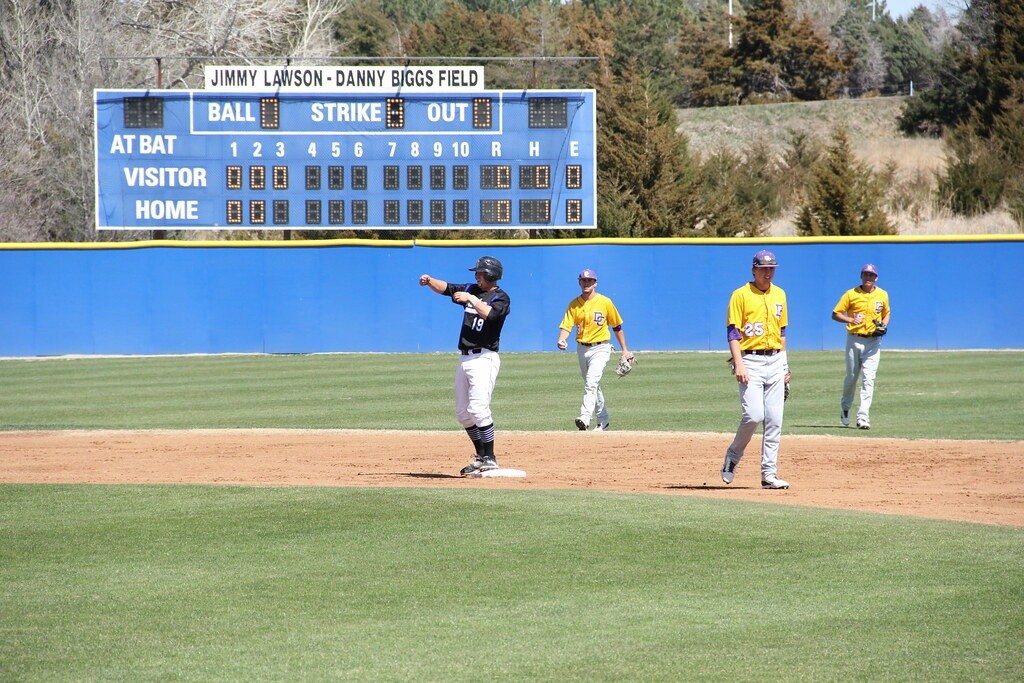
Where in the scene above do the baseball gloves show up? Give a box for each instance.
[613,351,636,378]
[784,381,791,403]
[871,318,887,337]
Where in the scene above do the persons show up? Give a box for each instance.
[419,256,510,478]
[557,268,635,431]
[831,264,891,430]
[720,249,792,490]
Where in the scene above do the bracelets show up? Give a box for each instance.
[474,300,480,306]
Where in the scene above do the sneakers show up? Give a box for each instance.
[841,409,850,425]
[721,453,736,484]
[761,476,789,489]
[857,418,870,429]
[460,455,484,475]
[480,456,499,472]
[593,422,609,431]
[575,416,589,431]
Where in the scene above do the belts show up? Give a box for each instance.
[744,349,780,356]
[578,342,601,346]
[462,349,481,355]
[851,333,872,338]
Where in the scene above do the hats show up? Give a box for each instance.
[861,264,878,277]
[577,269,597,285]
[752,250,779,268]
[469,256,502,282]
